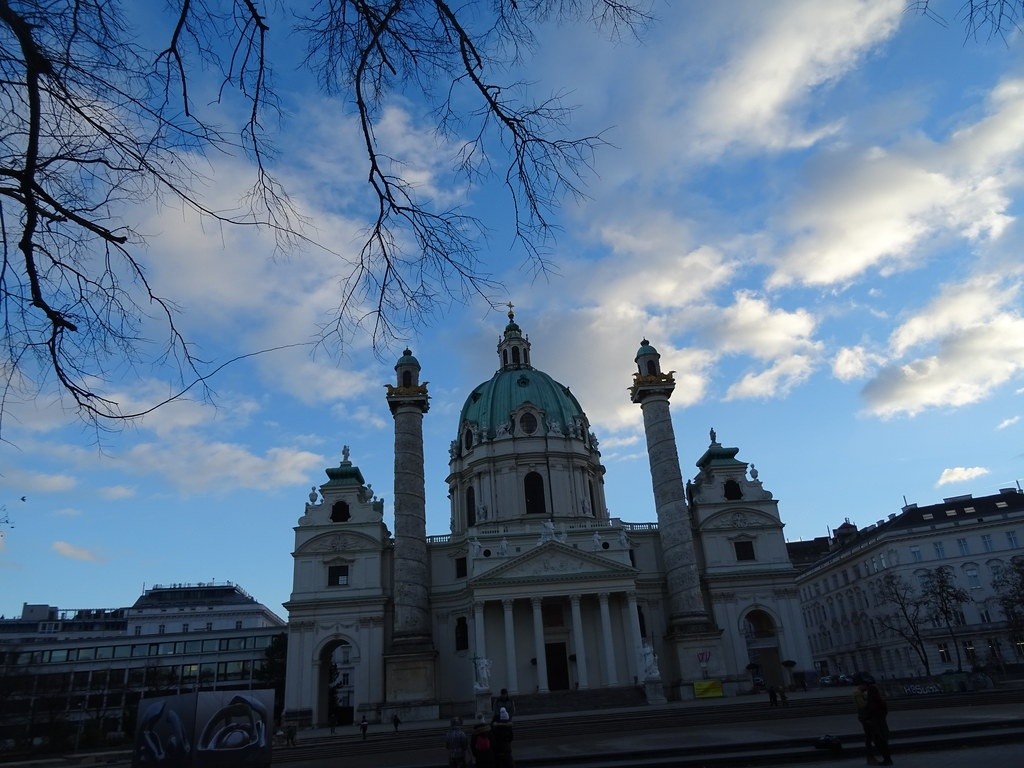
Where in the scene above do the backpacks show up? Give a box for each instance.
[475,735,489,750]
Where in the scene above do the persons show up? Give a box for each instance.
[768,686,777,707]
[393,715,401,732]
[492,688,514,722]
[329,714,336,735]
[286,721,296,746]
[470,713,497,768]
[360,716,368,740]
[801,679,809,692]
[445,716,467,768]
[852,671,893,766]
[491,706,514,768]
[779,683,791,707]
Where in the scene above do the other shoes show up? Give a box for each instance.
[867,756,893,767]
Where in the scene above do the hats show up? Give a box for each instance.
[451,717,461,726]
[472,713,485,725]
[499,707,509,720]
